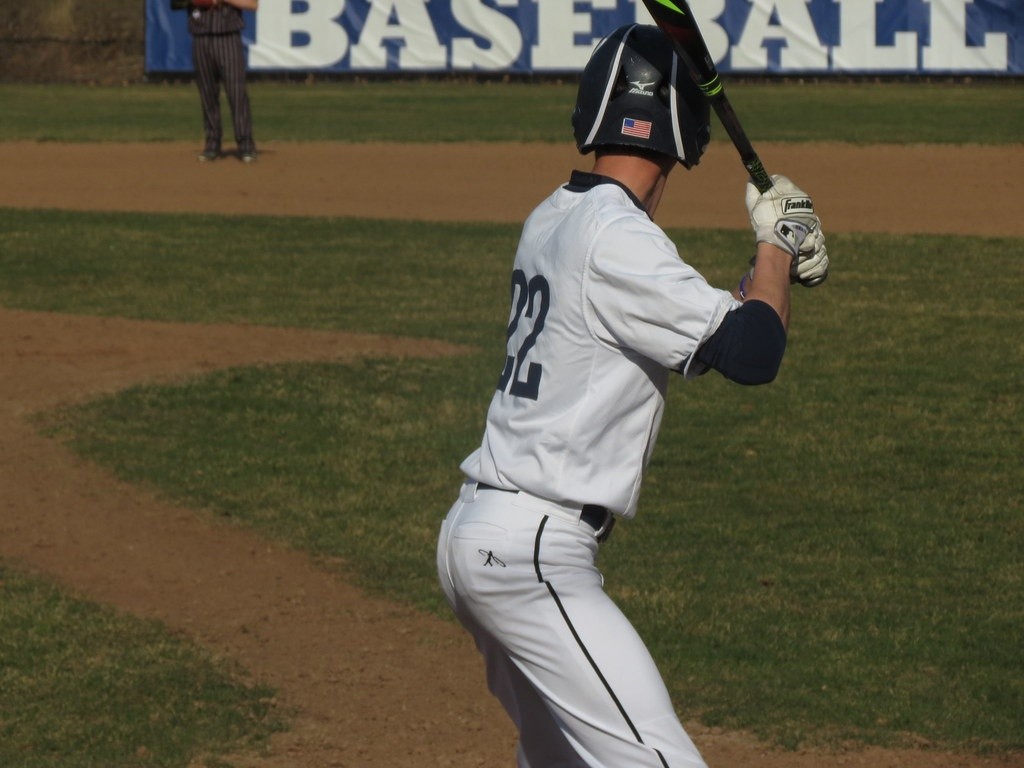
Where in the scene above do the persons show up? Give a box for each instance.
[426,14,835,767]
[173,1,269,164]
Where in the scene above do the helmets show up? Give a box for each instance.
[571,21,711,169]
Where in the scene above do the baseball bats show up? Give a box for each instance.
[643,0,775,196]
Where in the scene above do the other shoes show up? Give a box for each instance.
[238,149,257,162]
[199,150,221,162]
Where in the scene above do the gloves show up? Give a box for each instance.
[745,173,814,257]
[789,215,829,281]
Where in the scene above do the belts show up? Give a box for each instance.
[475,482,617,543]
